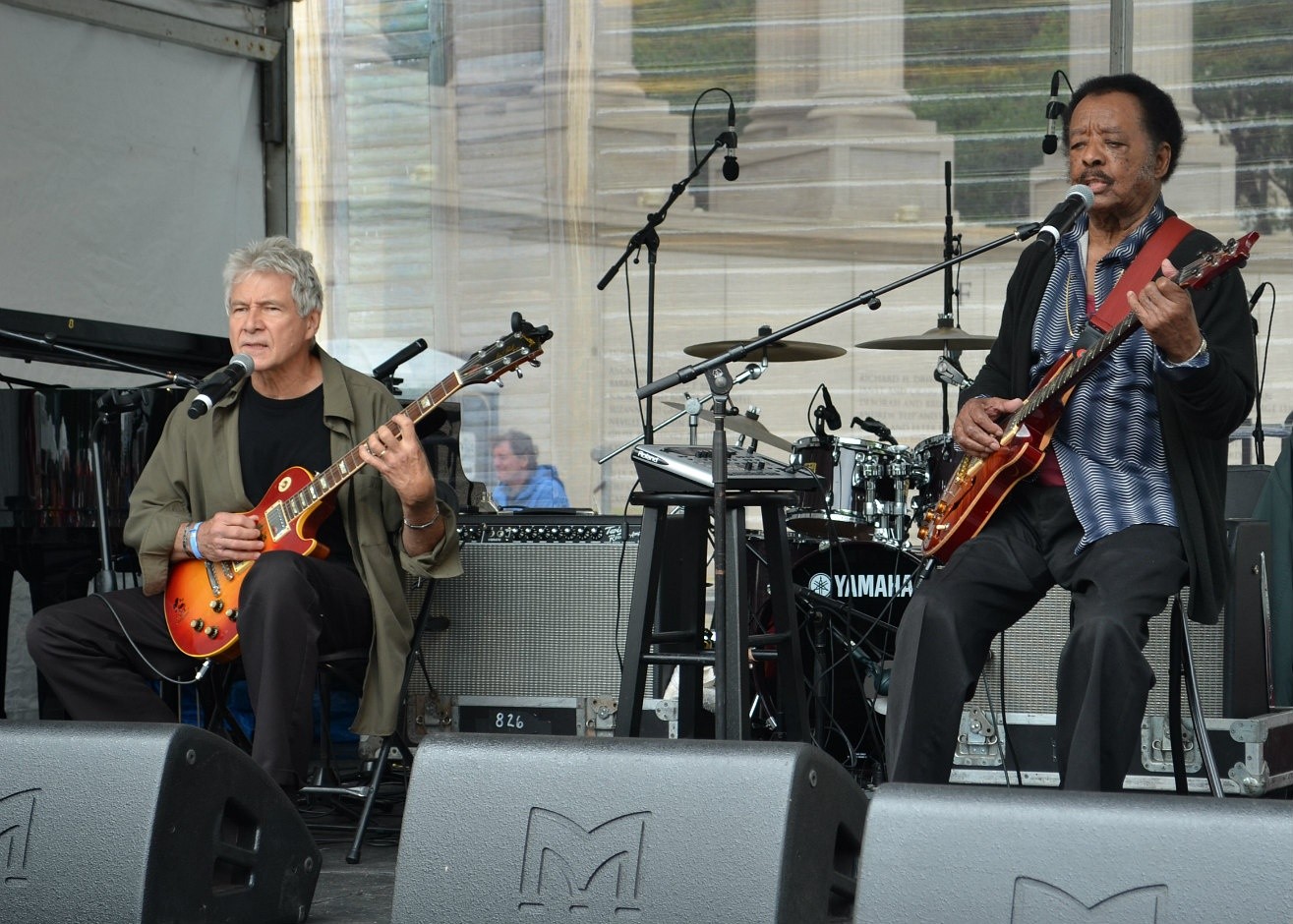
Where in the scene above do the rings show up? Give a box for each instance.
[378,449,387,457]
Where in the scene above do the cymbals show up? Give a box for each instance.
[855,327,999,350]
[683,336,847,362]
[661,401,794,453]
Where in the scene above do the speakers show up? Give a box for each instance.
[0,719,322,924]
[392,506,1293,924]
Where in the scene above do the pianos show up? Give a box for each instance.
[0,307,502,733]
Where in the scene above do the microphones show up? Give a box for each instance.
[822,386,842,430]
[1042,72,1060,155]
[1036,184,1095,254]
[1248,282,1266,312]
[872,668,892,695]
[723,102,741,181]
[185,352,255,418]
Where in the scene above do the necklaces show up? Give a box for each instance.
[1066,271,1075,337]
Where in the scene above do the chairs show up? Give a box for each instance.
[202,481,461,889]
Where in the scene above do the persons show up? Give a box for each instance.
[490,432,568,511]
[25,228,464,795]
[886,74,1257,790]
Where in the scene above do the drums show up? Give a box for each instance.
[912,433,966,529]
[710,529,823,634]
[785,436,912,541]
[750,541,926,759]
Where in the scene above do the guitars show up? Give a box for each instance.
[163,311,554,666]
[918,230,1261,566]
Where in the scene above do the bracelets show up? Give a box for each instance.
[1168,337,1206,365]
[404,504,439,529]
[183,523,192,557]
[189,522,205,559]
